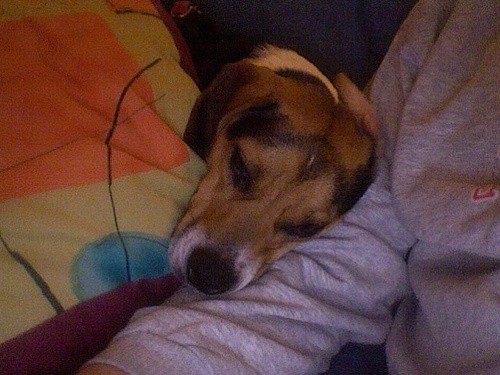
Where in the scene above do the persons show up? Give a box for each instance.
[75,0,500,373]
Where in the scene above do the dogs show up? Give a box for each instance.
[167,41,385,299]
